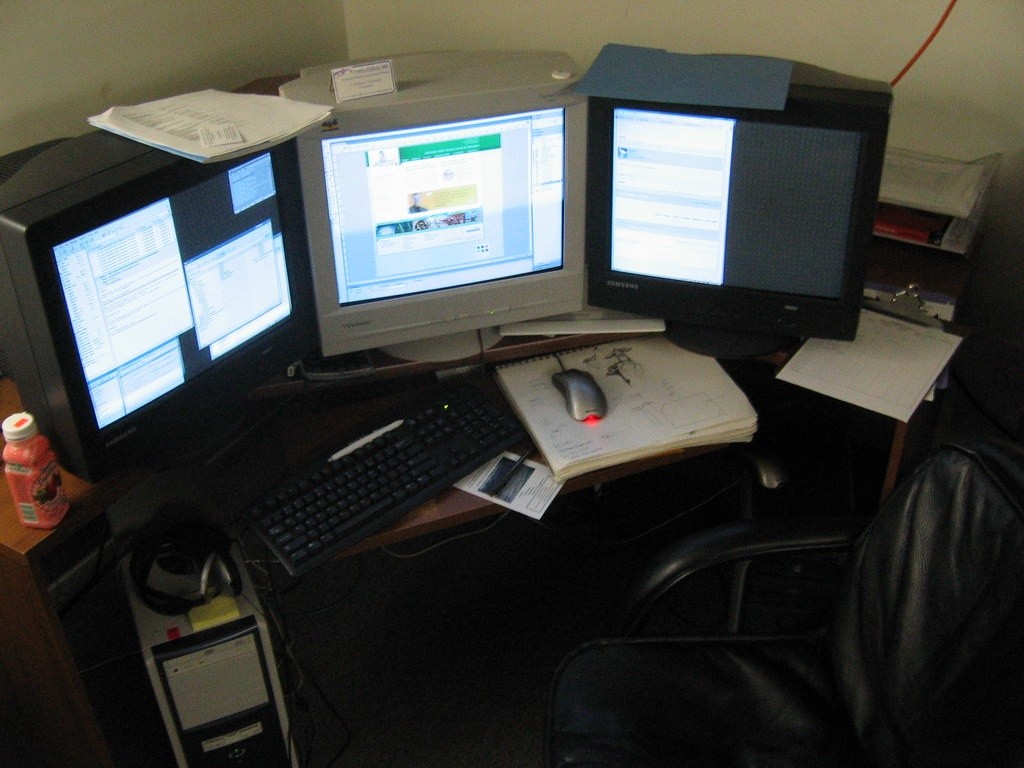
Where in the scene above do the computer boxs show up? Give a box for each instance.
[117,525,300,768]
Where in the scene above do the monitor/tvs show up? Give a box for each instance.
[276,48,588,362]
[0,129,318,486]
[584,55,894,361]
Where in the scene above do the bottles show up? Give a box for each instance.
[2,414,69,529]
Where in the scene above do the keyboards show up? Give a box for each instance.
[243,384,528,578]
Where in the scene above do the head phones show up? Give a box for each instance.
[128,525,244,617]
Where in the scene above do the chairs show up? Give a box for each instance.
[541,443,1024,768]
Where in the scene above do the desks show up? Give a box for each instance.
[0,306,919,768]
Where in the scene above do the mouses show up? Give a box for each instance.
[551,369,608,422]
